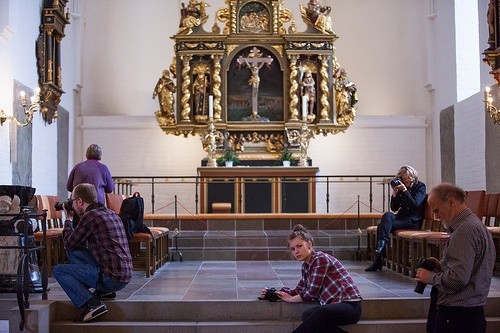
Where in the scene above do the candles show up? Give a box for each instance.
[302,96,307,117]
[209,95,213,118]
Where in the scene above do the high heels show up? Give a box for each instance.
[365,255,382,272]
[374,240,387,255]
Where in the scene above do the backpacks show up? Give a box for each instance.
[119,192,145,233]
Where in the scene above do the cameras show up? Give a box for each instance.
[55,198,73,211]
[390,177,404,188]
[414,258,436,295]
[260,288,282,302]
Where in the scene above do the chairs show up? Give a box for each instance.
[33,193,169,278]
[366,190,500,278]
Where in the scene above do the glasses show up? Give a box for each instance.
[69,197,81,206]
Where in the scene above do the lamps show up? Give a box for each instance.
[0,88,40,127]
[485,87,500,125]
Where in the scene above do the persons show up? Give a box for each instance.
[303,70,316,114]
[365,165,426,272]
[242,58,268,98]
[194,74,210,115]
[336,69,359,124]
[152,70,176,118]
[67,144,115,228]
[259,224,362,333]
[52,183,133,323]
[414,183,496,333]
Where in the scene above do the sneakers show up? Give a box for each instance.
[91,289,116,300]
[73,302,108,323]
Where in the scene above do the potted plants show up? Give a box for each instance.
[218,146,240,167]
[278,147,292,167]
